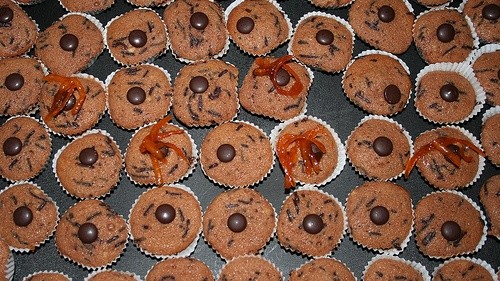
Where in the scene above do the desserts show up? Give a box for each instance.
[0,0,500,281]
